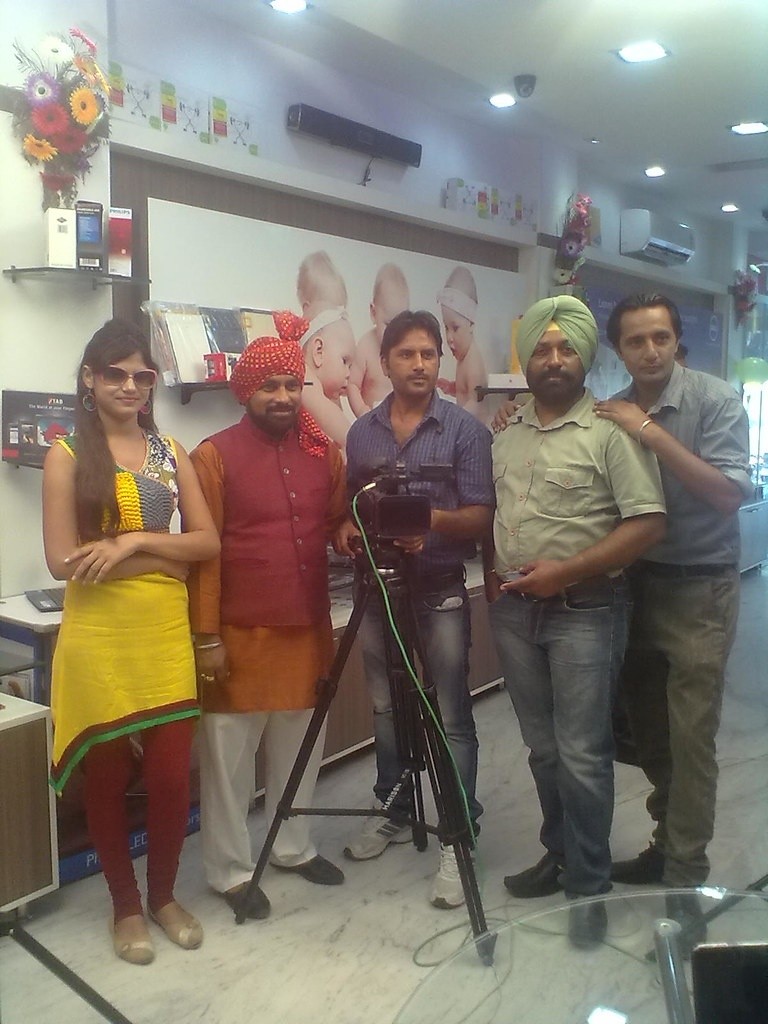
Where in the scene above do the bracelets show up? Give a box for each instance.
[196,642,223,649]
[638,419,653,448]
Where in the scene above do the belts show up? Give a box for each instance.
[497,577,564,602]
[634,560,733,577]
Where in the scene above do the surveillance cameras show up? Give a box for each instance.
[515,74,537,98]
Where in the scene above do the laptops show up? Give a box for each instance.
[25,588,67,612]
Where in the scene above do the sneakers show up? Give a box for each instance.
[430,836,480,909]
[345,798,414,860]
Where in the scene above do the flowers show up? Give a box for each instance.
[10,26,112,212]
[553,192,593,284]
[728,268,763,327]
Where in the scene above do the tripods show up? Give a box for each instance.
[234,533,499,966]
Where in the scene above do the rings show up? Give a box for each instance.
[201,673,214,681]
[227,672,231,679]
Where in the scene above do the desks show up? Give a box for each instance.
[0,588,65,705]
[393,887,768,1024]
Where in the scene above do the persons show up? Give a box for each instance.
[481,295,668,949]
[436,266,489,424]
[297,251,410,449]
[185,337,348,920]
[490,294,755,959]
[331,309,497,910]
[41,317,222,965]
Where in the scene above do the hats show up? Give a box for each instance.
[516,296,599,380]
[229,312,331,457]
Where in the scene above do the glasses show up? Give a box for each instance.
[100,363,158,390]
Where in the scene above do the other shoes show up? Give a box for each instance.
[503,865,565,898]
[268,856,345,884]
[570,894,607,947]
[222,880,269,918]
[147,897,202,949]
[609,851,664,885]
[114,914,155,964]
[666,887,707,959]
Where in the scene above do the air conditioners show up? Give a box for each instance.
[620,208,696,266]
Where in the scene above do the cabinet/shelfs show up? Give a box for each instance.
[0,692,61,919]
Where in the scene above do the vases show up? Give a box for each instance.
[548,285,576,297]
